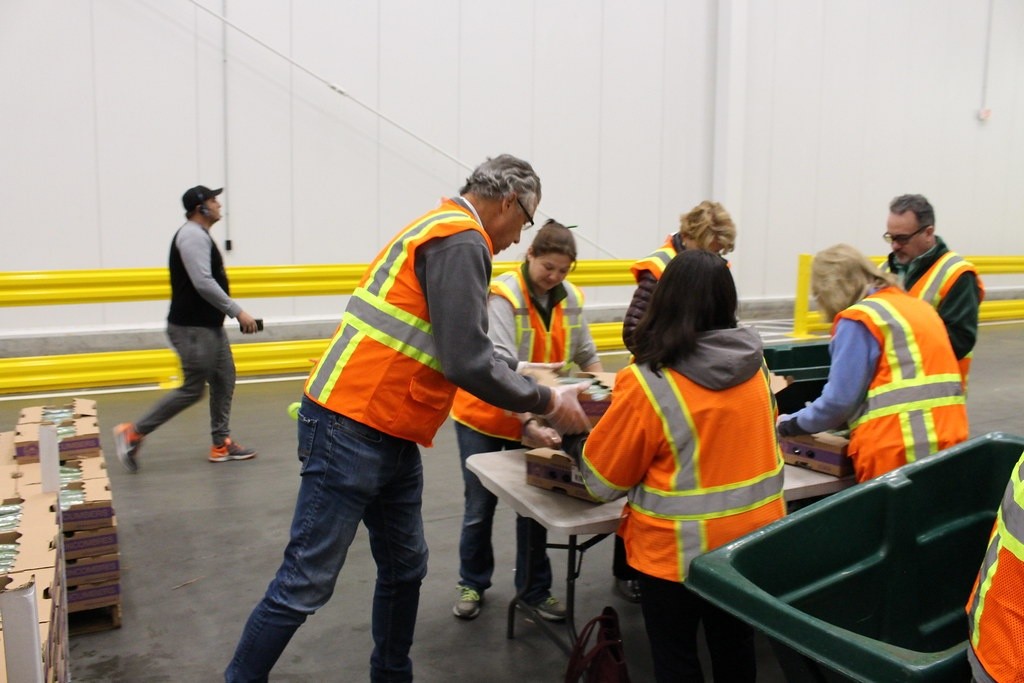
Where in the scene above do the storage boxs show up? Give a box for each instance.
[764,340,831,415]
[524,447,601,504]
[0,398,123,683]
[778,431,855,478]
[539,372,617,428]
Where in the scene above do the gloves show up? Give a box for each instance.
[561,431,589,466]
[778,416,809,438]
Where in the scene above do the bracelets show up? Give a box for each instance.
[536,386,563,419]
[522,416,537,431]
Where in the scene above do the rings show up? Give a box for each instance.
[551,437,558,441]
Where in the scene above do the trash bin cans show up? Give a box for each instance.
[687,427,1024,683]
[758,336,834,414]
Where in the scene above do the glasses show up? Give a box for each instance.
[883,224,932,244]
[517,198,534,230]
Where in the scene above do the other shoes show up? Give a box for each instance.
[615,577,642,603]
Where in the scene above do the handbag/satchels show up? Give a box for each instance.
[567,605,628,683]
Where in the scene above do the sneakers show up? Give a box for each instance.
[515,596,566,621]
[208,435,257,462]
[453,585,482,618]
[111,423,147,475]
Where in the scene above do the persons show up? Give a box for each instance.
[776,243,970,487]
[613,202,738,601]
[450,220,602,621]
[113,185,264,471]
[580,249,790,683]
[966,443,1023,681]
[221,154,594,683]
[873,194,987,410]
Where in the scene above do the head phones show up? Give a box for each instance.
[192,187,210,216]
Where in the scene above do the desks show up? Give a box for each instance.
[464,448,859,681]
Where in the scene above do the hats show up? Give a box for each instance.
[182,185,222,212]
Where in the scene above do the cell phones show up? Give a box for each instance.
[240,319,263,331]
